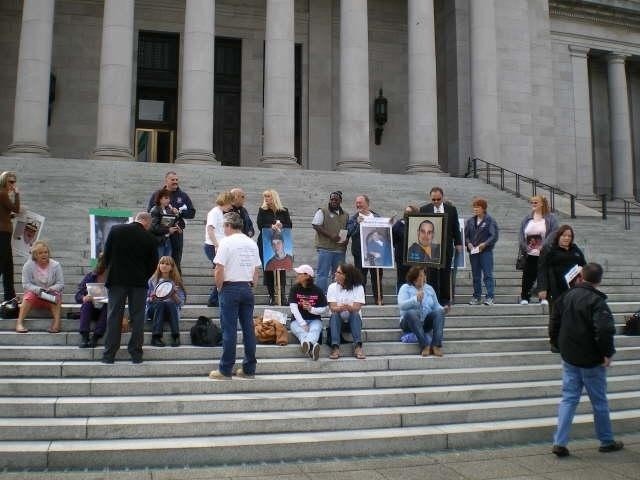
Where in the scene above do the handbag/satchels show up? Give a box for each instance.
[516,255,528,269]
[0,298,19,319]
[191,316,222,347]
[625,309,640,336]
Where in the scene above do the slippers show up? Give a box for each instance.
[48,327,60,333]
[16,328,28,332]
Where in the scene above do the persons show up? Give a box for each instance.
[548,263,624,456]
[348,195,396,305]
[367,229,392,266]
[203,189,293,378]
[0,171,20,301]
[408,220,440,262]
[397,264,448,356]
[465,196,498,305]
[75,170,196,365]
[311,190,349,294]
[16,241,65,332]
[392,205,418,293]
[419,186,462,308]
[288,265,330,361]
[520,195,560,305]
[327,264,366,359]
[537,225,588,353]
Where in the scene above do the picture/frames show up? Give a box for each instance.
[154,279,176,301]
[402,213,448,269]
[160,213,177,238]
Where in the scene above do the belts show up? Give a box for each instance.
[223,281,252,286]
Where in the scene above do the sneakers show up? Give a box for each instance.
[309,342,320,361]
[482,297,496,305]
[267,295,274,305]
[353,348,365,359]
[422,345,432,356]
[329,350,341,359]
[79,335,89,348]
[551,344,559,353]
[88,336,99,347]
[282,294,290,306]
[101,359,114,362]
[236,368,256,379]
[433,346,443,355]
[551,445,568,455]
[207,302,218,307]
[301,340,313,357]
[133,359,145,363]
[468,297,482,305]
[209,370,232,380]
[540,298,549,304]
[599,441,624,452]
[520,299,528,304]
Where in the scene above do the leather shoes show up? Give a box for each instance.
[171,337,180,347]
[151,335,165,347]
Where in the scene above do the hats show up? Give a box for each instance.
[292,264,314,278]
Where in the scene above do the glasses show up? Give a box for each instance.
[330,197,339,201]
[8,181,16,183]
[431,197,441,201]
[336,270,345,275]
[241,196,245,198]
[530,202,539,204]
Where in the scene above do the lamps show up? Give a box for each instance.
[374,87,388,143]
